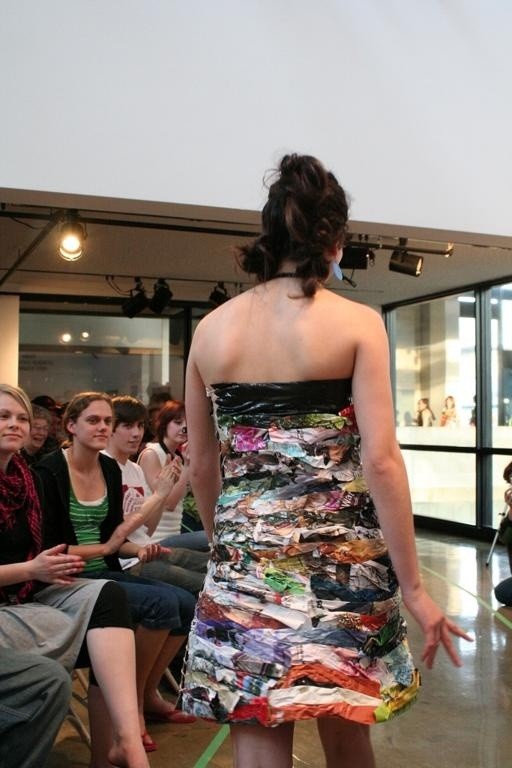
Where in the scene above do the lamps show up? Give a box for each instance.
[123,279,230,320]
[55,207,88,264]
[57,319,185,356]
[341,235,424,277]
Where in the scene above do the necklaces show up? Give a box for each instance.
[268,268,311,279]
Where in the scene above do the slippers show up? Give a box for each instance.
[143,709,197,724]
[141,732,157,752]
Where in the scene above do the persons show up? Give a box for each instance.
[438,395,458,429]
[173,149,477,767]
[492,459,512,608]
[416,397,435,427]
[1,381,211,767]
